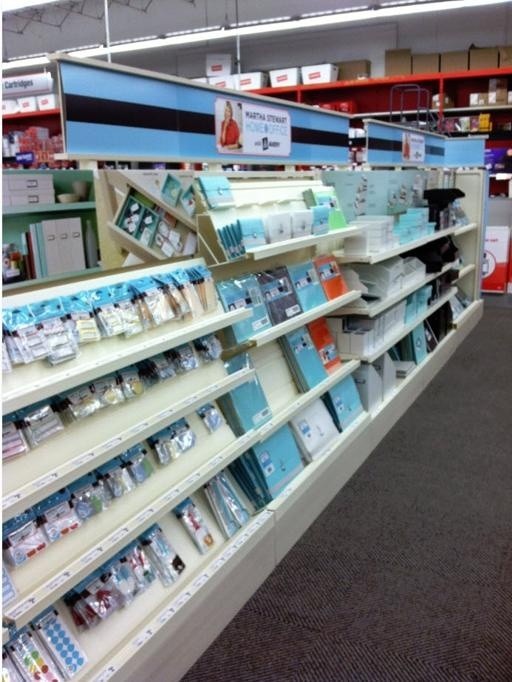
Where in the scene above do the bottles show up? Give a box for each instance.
[85,220,99,267]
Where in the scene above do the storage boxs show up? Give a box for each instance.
[301,44,512,86]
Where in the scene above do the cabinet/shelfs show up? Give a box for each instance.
[1,67,512,682]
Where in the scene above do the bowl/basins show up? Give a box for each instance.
[58,194,79,202]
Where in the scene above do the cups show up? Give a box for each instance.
[71,180,89,200]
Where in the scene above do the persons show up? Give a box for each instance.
[219,100,240,149]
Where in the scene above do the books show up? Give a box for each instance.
[215,254,367,512]
